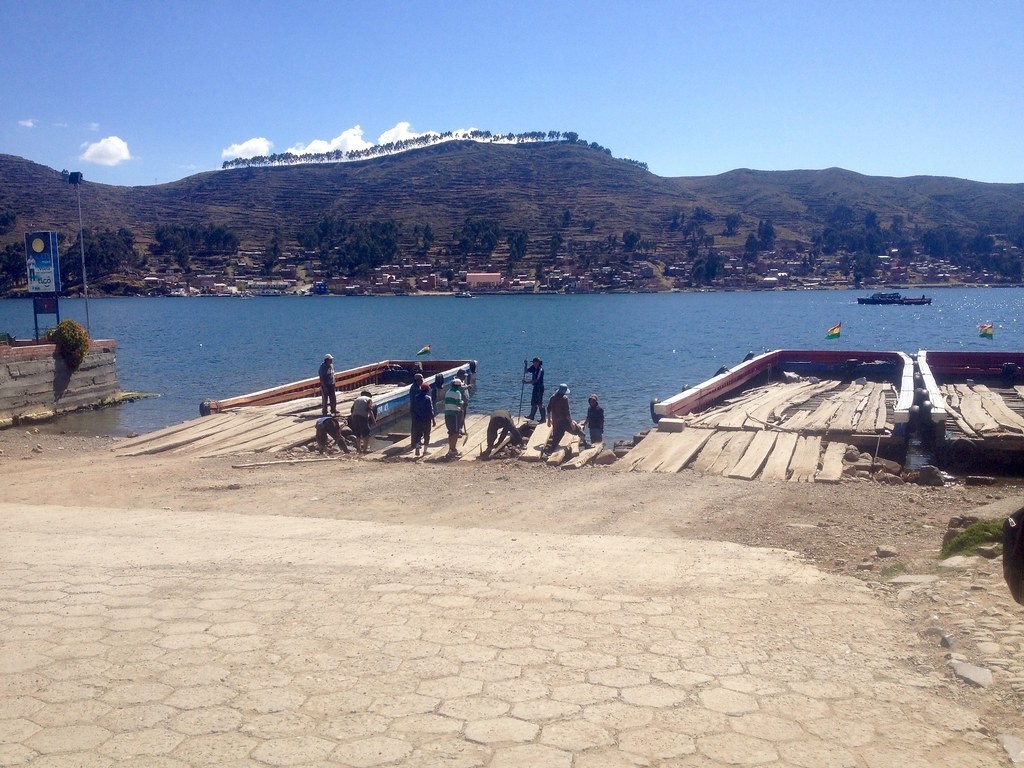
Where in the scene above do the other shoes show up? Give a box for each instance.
[520,444,527,449]
[481,450,491,457]
[448,449,461,456]
[459,430,467,438]
[342,447,351,453]
[546,447,553,455]
[584,442,594,448]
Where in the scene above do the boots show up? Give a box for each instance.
[539,407,546,422]
[524,405,536,420]
[416,442,420,455]
[424,445,431,455]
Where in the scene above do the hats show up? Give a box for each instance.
[420,383,432,391]
[556,383,571,394]
[324,354,334,360]
[530,358,542,363]
[457,369,469,377]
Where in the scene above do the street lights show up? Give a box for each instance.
[68,171,90,339]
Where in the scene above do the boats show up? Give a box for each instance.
[454,292,472,298]
[856,292,932,305]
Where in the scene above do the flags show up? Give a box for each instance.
[825,324,840,340]
[979,325,994,340]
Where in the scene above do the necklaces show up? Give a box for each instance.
[445,379,464,458]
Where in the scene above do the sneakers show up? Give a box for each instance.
[357,448,372,453]
[322,410,341,414]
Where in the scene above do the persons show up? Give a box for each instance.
[482,409,524,455]
[546,383,596,456]
[452,368,474,438]
[351,391,376,452]
[410,374,436,455]
[522,358,546,423]
[582,394,604,443]
[318,354,340,417]
[315,417,351,454]
